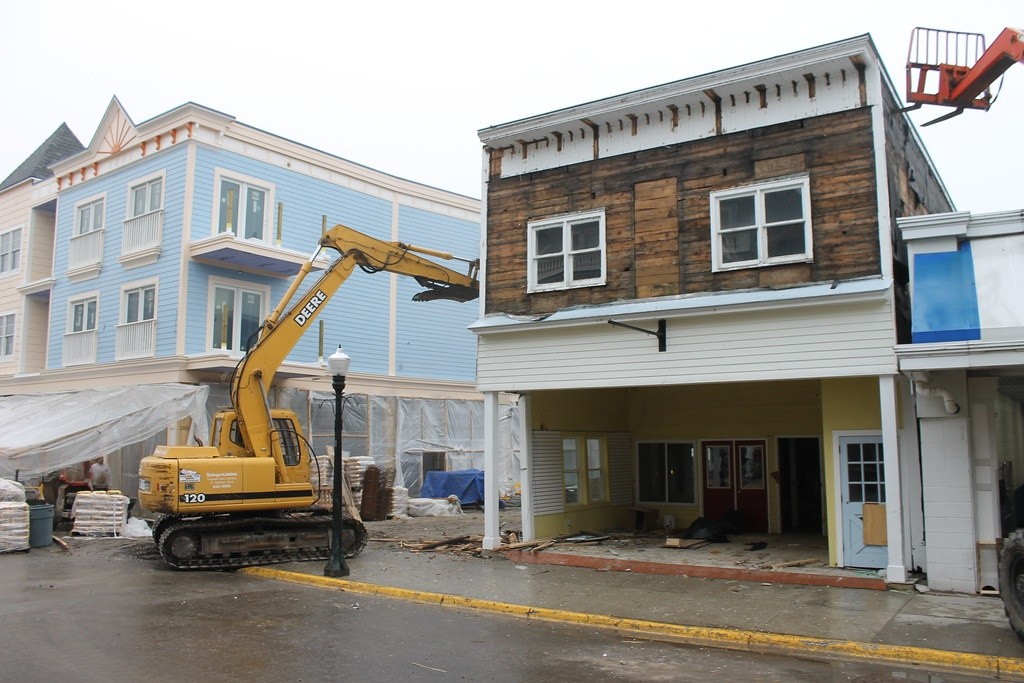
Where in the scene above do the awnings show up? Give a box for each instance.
[0,383,210,481]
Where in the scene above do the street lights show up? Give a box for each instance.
[323,341,349,581]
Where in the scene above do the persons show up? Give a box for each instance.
[88,457,112,491]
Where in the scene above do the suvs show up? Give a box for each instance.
[906,23,1024,638]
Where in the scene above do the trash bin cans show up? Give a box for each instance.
[28,504,55,547]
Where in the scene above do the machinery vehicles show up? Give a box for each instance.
[135,223,481,573]
